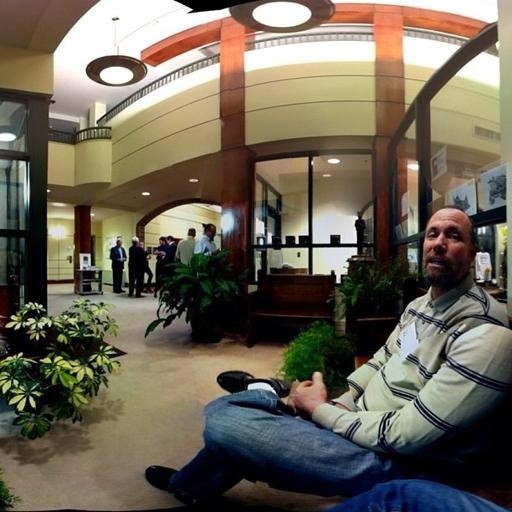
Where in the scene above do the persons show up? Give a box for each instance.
[110,240,128,293]
[144,206,512,507]
[323,478,512,512]
[128,224,216,298]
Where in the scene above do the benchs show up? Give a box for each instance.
[355,276,430,369]
[250,271,336,331]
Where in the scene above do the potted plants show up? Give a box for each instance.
[144,248,250,344]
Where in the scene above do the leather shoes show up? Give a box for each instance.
[217,371,282,395]
[145,465,192,506]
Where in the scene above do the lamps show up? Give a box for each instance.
[229,0,336,33]
[84,18,147,86]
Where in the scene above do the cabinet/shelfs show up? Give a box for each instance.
[74,269,103,295]
